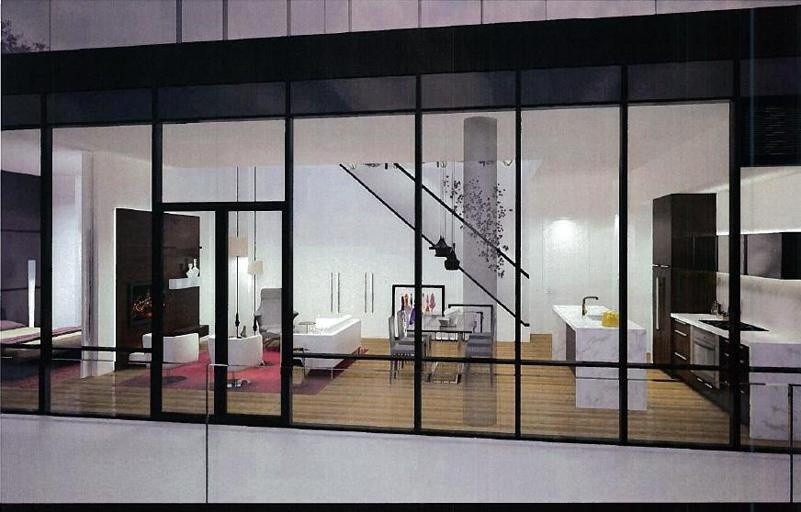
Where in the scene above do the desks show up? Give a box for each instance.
[297,319,317,332]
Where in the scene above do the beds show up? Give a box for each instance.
[0,317,82,365]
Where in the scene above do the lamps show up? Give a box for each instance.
[229,231,250,339]
[248,259,264,333]
[428,161,465,272]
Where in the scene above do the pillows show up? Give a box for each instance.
[0,318,25,330]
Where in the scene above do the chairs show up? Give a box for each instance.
[207,333,264,391]
[147,333,201,385]
[254,286,299,351]
[387,307,498,384]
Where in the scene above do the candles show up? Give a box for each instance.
[390,283,447,340]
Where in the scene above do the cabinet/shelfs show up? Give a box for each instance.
[650,192,720,379]
[718,336,752,428]
[672,319,689,384]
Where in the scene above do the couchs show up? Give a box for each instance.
[278,313,363,381]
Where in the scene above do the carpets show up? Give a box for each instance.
[113,343,367,398]
[0,355,87,391]
